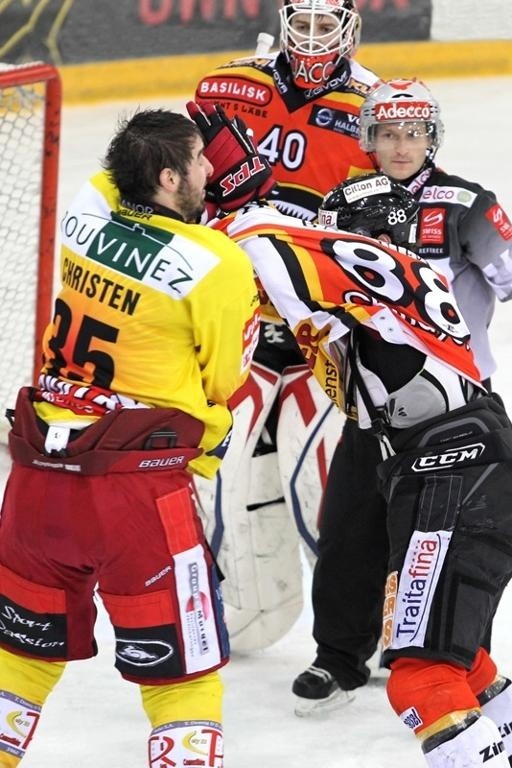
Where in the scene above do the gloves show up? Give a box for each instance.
[181,97,276,207]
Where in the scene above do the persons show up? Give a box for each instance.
[183,97,512,768]
[285,77,508,697]
[0,107,265,768]
[184,0,388,627]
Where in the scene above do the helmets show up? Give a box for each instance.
[357,75,446,153]
[279,0,363,88]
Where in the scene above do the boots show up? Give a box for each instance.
[294,656,352,702]
